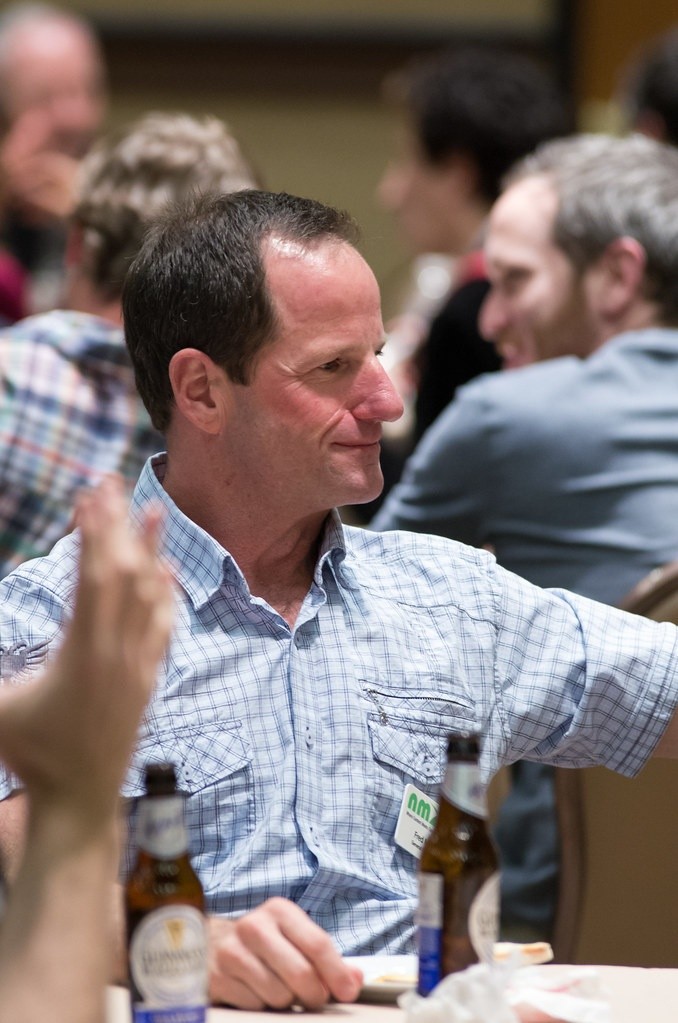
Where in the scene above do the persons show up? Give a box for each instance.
[0,1,678,1023]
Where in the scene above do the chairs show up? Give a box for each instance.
[550,560,678,968]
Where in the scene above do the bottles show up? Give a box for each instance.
[123,763,213,1023]
[417,729,499,998]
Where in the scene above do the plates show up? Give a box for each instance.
[343,955,417,1002]
